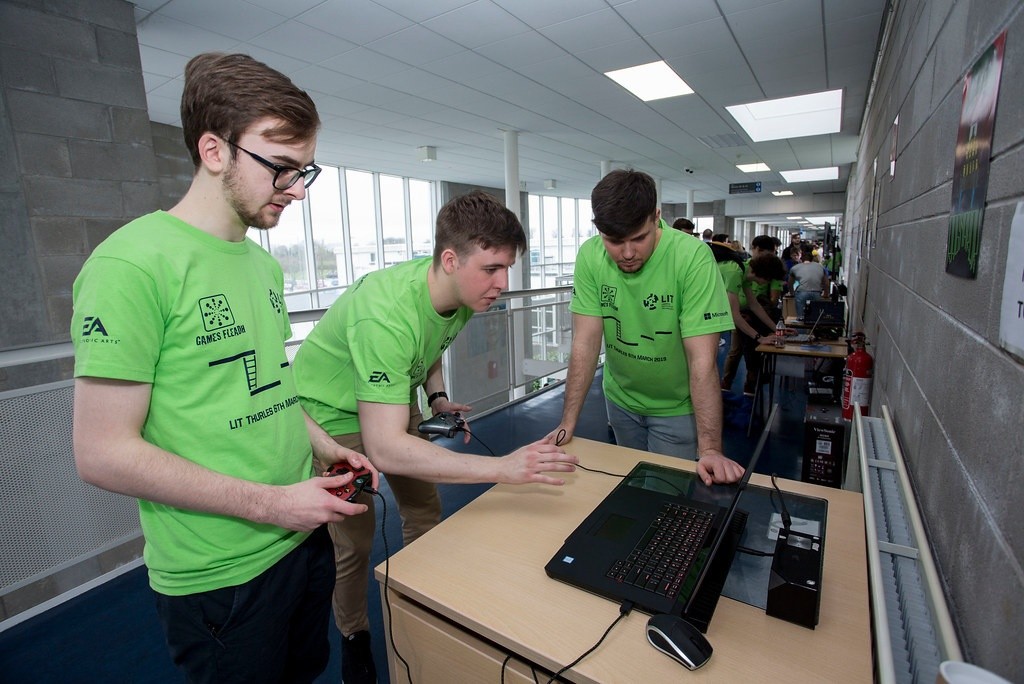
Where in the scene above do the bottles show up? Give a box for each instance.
[775,316,786,348]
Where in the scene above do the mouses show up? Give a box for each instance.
[647,614,714,671]
[773,341,784,348]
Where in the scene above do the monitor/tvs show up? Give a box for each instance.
[823,222,831,257]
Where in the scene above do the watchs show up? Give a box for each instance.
[754,334,762,341]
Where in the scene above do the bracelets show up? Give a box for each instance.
[428,392,448,407]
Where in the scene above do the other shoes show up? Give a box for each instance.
[720,380,732,393]
[744,384,754,397]
[718,338,726,347]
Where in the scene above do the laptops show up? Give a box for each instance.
[783,309,825,343]
[545,403,779,616]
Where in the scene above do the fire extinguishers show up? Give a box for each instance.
[842,331,874,420]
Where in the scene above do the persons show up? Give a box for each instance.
[672,218,842,397]
[542,169,746,486]
[290,189,579,684]
[71,52,379,684]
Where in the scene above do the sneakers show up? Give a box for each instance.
[341,630,381,684]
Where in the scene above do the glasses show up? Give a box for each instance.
[223,138,322,190]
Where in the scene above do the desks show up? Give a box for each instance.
[373,438,873,684]
[782,291,832,320]
[746,316,849,438]
